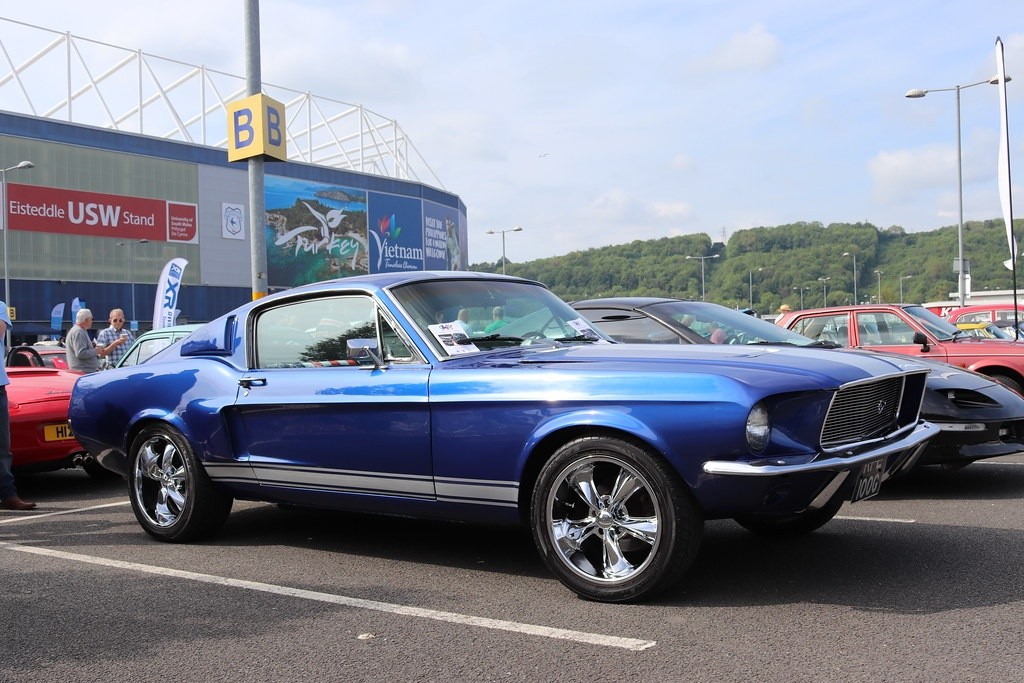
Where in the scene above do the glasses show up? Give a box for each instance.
[112,318,122,322]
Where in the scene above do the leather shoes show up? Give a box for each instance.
[0,497,37,509]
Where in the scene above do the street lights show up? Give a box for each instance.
[817,276,831,308]
[904,74,1014,309]
[899,275,912,304]
[874,269,883,304]
[686,254,721,302]
[485,225,523,276]
[0,159,35,355]
[794,287,810,311]
[842,252,857,306]
[748,268,764,310]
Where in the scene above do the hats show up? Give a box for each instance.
[777,305,792,312]
[60,336,65,340]
[743,310,756,314]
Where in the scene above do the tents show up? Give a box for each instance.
[11,323,62,336]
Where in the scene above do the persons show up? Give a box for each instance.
[0,301,38,510]
[485,306,510,333]
[65,309,105,373]
[455,308,473,338]
[444,214,460,271]
[57,336,65,348]
[774,304,800,332]
[96,309,134,366]
[707,321,726,344]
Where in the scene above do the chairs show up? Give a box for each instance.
[836,325,876,345]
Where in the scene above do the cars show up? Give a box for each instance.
[5,345,121,482]
[772,302,1024,399]
[483,296,1024,489]
[104,322,209,371]
[67,267,944,605]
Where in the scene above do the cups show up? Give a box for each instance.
[121,334,127,339]
[96,343,104,348]
